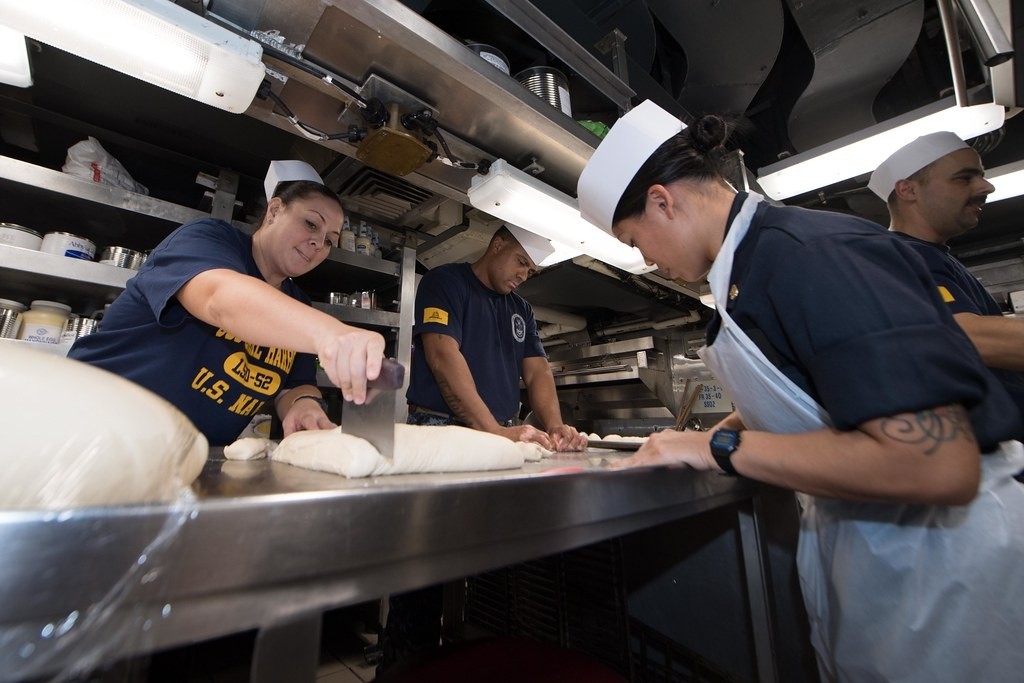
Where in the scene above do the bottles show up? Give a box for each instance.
[332,215,382,259]
[0,297,111,346]
[329,288,377,310]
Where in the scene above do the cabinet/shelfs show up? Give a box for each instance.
[1,153,417,424]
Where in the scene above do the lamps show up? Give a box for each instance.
[756,44,1024,204]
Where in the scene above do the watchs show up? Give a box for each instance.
[293,395,332,421]
[709,427,741,477]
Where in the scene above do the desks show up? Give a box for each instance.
[0,438,783,683]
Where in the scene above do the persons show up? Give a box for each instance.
[61,157,386,459]
[375,221,589,682]
[869,131,1024,484]
[577,99,1024,683]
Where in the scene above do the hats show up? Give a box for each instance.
[867,131,972,203]
[577,99,689,238]
[505,222,558,266]
[264,160,324,203]
